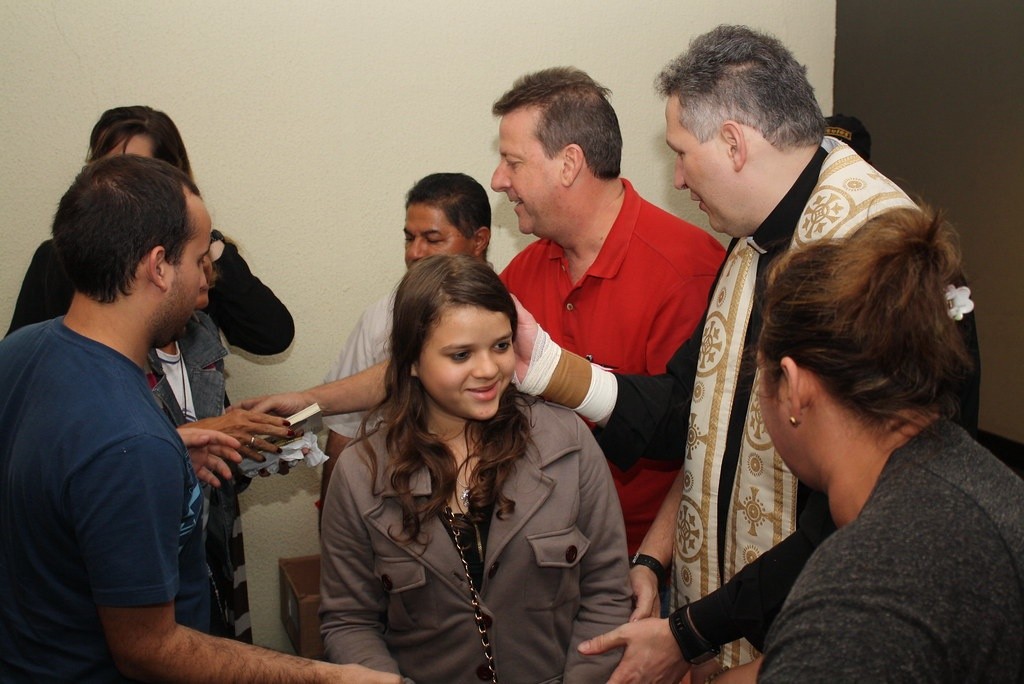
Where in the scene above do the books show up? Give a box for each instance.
[247,401,323,453]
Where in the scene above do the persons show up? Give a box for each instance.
[577,24,981,684]
[4,102,294,638]
[313,173,491,535]
[222,65,729,620]
[0,157,406,684]
[715,210,1024,684]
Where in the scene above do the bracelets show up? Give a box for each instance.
[629,552,667,591]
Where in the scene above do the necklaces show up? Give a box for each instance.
[458,478,470,507]
[179,355,187,416]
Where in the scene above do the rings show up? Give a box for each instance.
[250,437,254,444]
